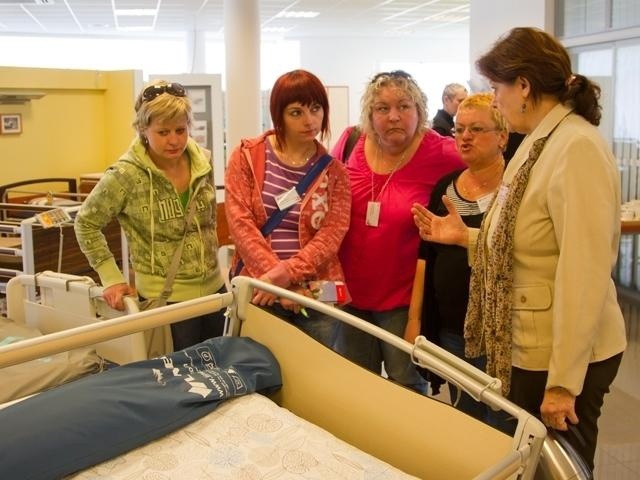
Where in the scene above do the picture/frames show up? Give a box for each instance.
[0,113,21,134]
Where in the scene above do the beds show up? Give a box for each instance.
[0,272,147,407]
[0,276,547,480]
[0,178,90,219]
[0,203,121,297]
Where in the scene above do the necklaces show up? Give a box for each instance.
[282,148,315,165]
[462,160,504,197]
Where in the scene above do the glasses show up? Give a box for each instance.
[142,83,187,101]
[371,70,411,83]
[451,126,500,135]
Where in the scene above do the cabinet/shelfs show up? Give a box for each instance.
[149,74,226,200]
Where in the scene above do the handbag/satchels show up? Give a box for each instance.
[229,154,333,284]
[133,149,211,358]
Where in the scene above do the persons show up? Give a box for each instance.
[224,70,352,350]
[412,27,624,476]
[403,94,513,436]
[329,71,469,396]
[424,83,468,151]
[73,79,227,354]
